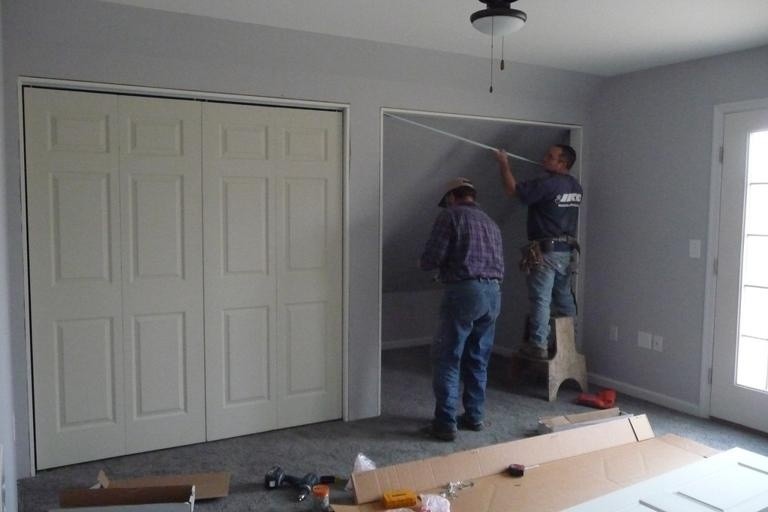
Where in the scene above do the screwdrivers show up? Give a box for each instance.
[319,476,348,485]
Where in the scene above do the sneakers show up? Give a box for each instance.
[518,346,549,362]
[458,413,484,431]
[421,424,457,440]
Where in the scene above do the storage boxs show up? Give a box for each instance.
[57,482,199,511]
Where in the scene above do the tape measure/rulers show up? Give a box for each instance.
[508,463,525,477]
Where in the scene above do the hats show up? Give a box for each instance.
[438,176,476,207]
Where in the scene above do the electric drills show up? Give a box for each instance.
[266,466,318,502]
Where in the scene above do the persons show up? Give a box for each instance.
[418,174,505,440]
[494,142,583,361]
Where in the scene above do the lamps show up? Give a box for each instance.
[466,0,528,96]
